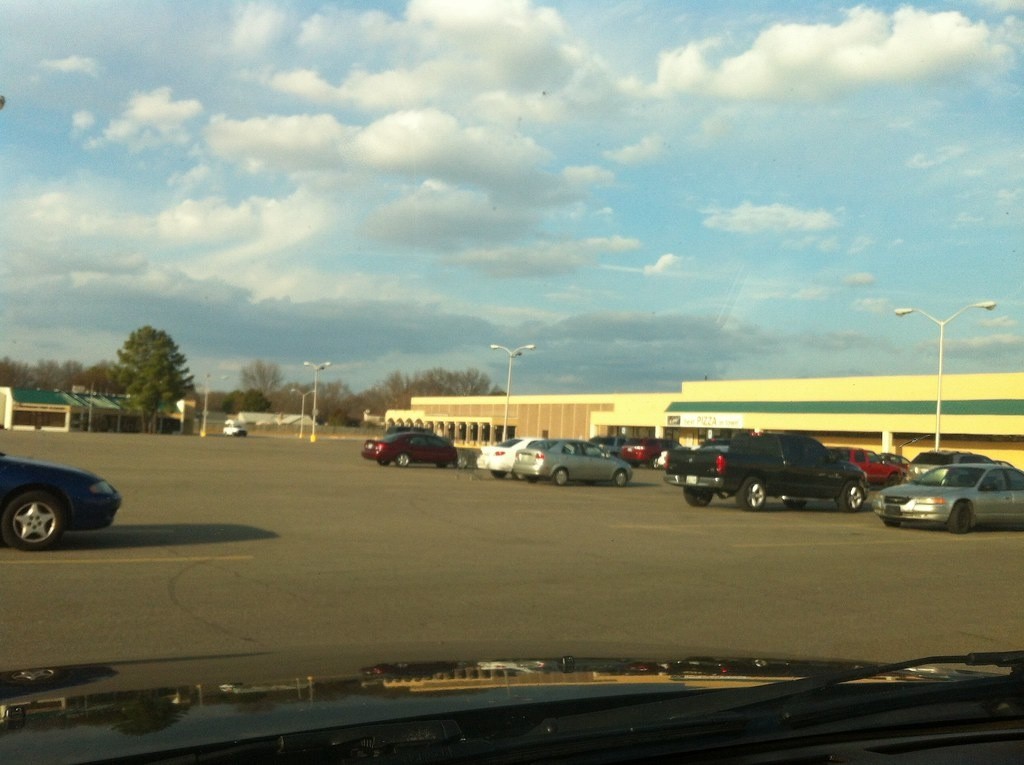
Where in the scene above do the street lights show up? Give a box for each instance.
[200,372,229,438]
[291,388,316,438]
[304,361,331,442]
[489,343,538,442]
[894,301,1000,454]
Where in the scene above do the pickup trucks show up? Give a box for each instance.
[664,432,870,513]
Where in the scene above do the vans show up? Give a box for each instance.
[223,420,248,437]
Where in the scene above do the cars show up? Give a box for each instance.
[873,462,1024,533]
[476,438,573,481]
[513,438,634,486]
[0,453,124,552]
[361,432,469,470]
[878,454,911,475]
[591,435,626,458]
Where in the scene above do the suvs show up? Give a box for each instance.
[387,426,430,437]
[903,451,998,485]
[831,447,903,488]
[622,437,683,468]
